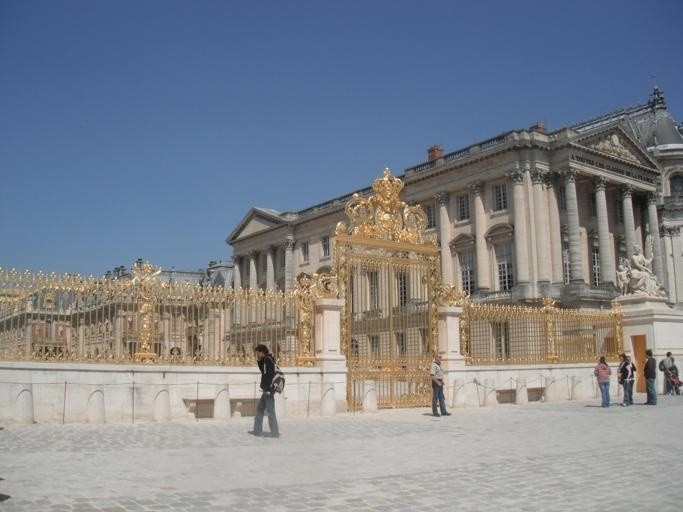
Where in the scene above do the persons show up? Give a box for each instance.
[247,343,280,439]
[643,349,657,406]
[662,351,673,396]
[670,356,681,396]
[429,351,452,418]
[628,244,655,289]
[617,352,632,407]
[626,355,637,404]
[593,354,612,407]
[616,258,631,296]
